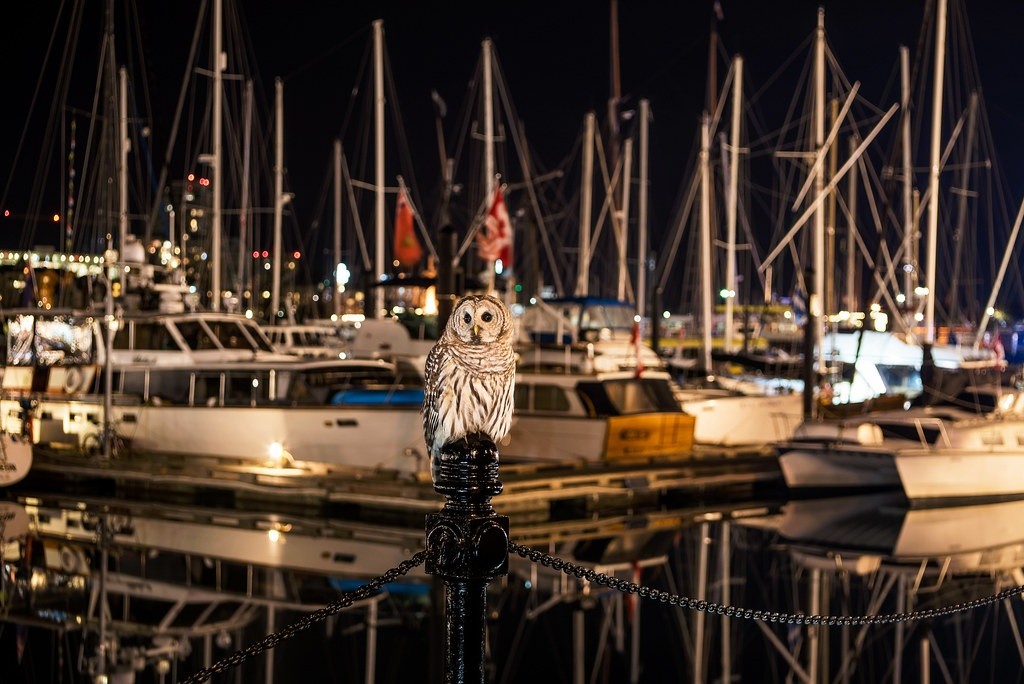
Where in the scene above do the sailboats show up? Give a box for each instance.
[1,1,1023,684]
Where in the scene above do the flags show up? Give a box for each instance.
[395,193,424,269]
[484,189,513,266]
